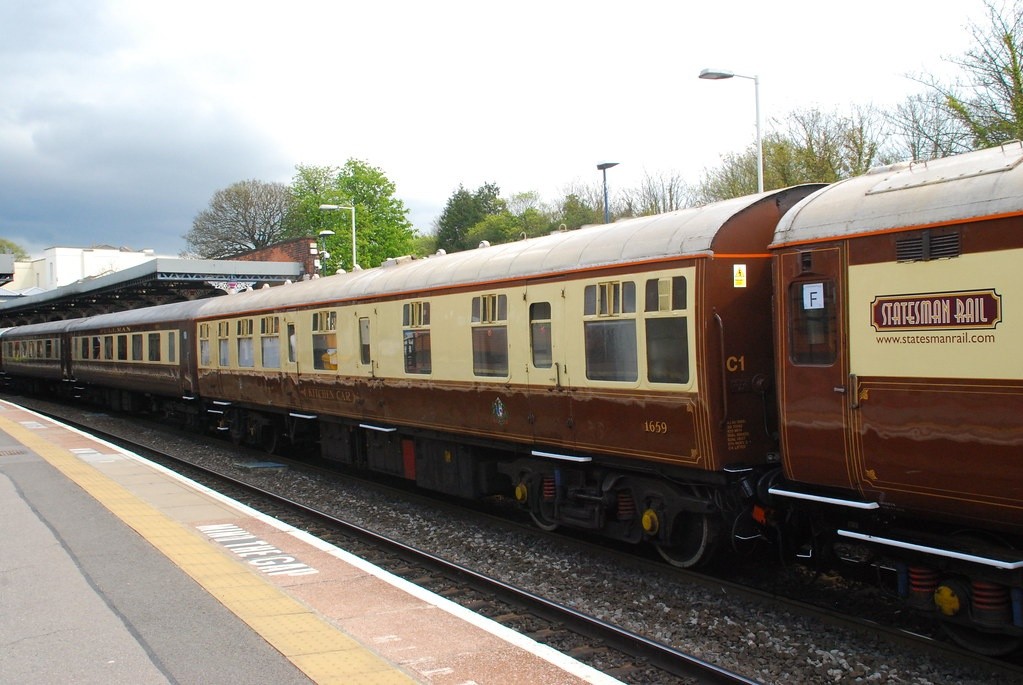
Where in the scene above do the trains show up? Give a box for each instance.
[0,139,1022,655]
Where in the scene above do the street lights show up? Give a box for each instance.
[319,230,334,277]
[698,68,764,194]
[596,163,618,223]
[319,205,357,268]
